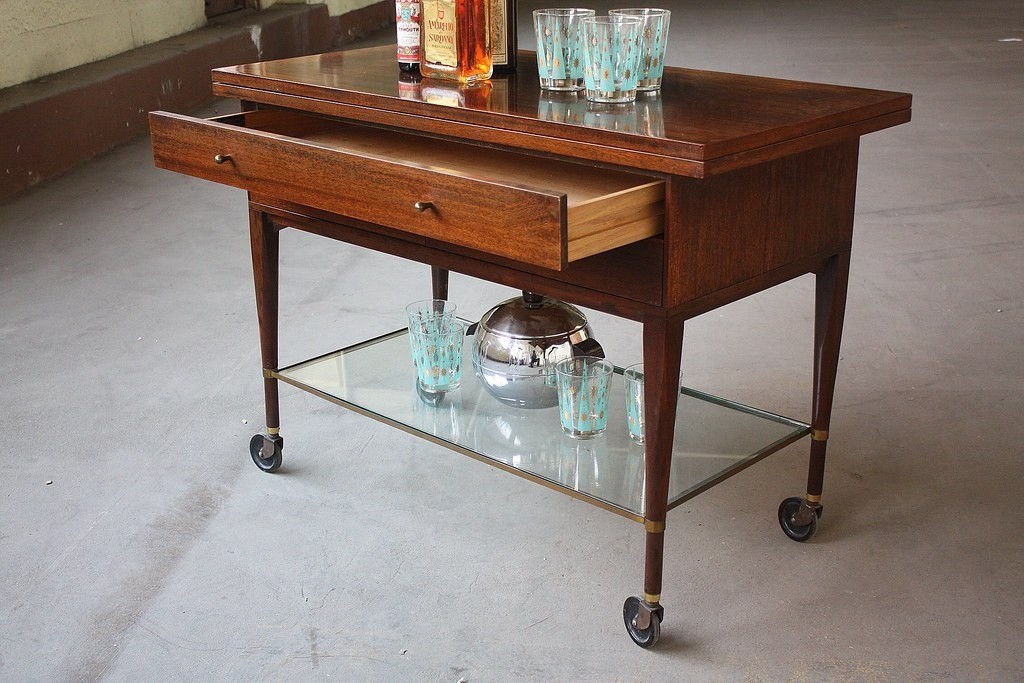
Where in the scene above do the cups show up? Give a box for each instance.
[580,16,646,103]
[625,363,684,446]
[410,318,465,393]
[533,8,595,90]
[406,299,457,366]
[554,356,614,440]
[609,8,671,90]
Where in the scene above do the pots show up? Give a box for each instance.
[466,289,606,408]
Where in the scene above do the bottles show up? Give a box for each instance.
[419,0,493,83]
[490,0,517,79]
[396,0,420,73]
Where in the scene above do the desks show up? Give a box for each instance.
[146,44,912,648]
